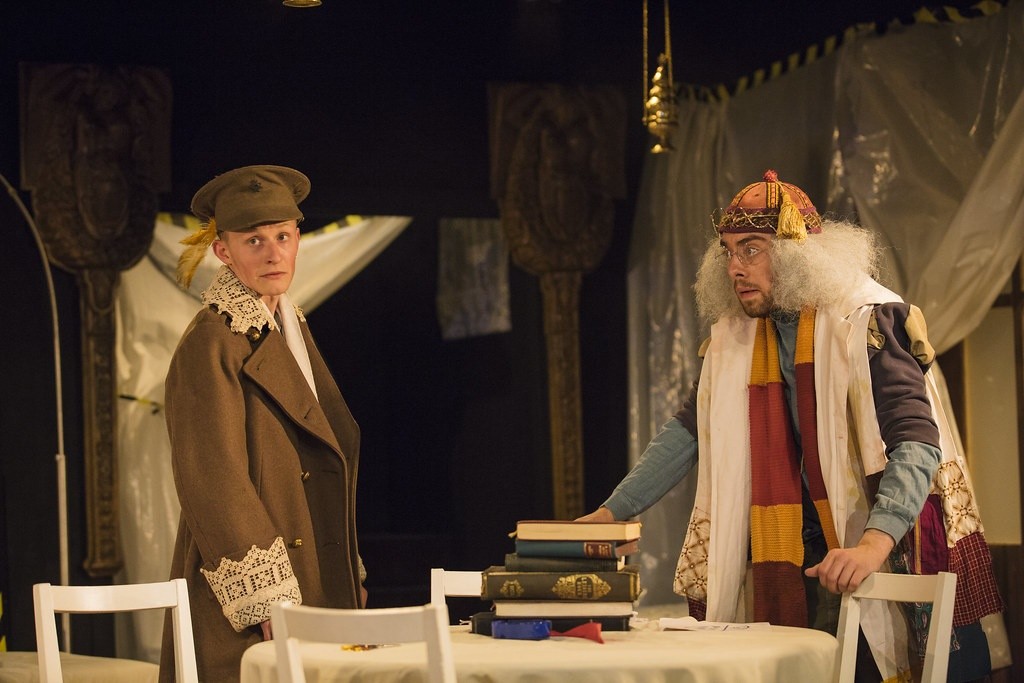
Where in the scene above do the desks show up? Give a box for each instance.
[241,616,835,683]
[0,649,160,683]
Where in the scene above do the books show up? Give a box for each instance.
[472,521,643,636]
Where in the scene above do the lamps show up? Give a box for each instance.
[642,0,680,154]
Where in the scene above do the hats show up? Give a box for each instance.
[718,170,822,241]
[191,165,311,232]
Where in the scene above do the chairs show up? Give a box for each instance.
[270,567,486,683]
[827,567,957,683]
[33,578,201,683]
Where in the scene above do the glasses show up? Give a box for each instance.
[714,242,774,267]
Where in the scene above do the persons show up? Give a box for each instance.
[576,169,943,683]
[157,165,367,683]
